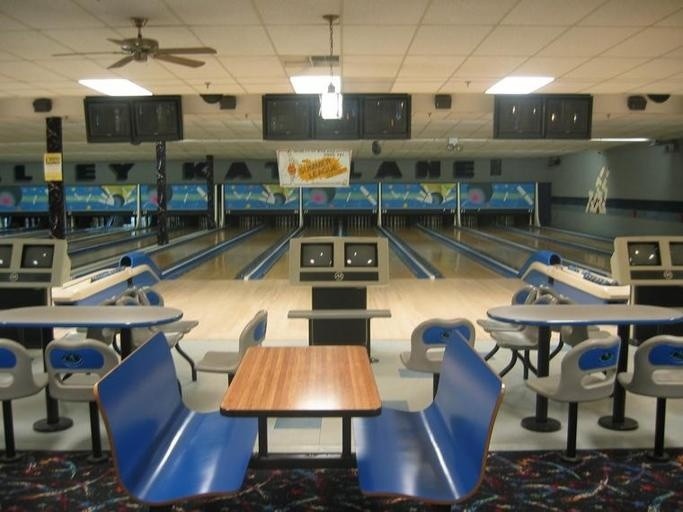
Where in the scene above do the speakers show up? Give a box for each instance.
[33,98,51,112]
[219,96,236,108]
[435,95,451,109]
[628,97,646,109]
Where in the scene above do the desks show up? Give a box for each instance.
[491,304,683,433]
[1,304,182,432]
[220,345,385,471]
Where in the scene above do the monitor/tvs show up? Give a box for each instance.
[0,244,13,269]
[628,242,662,265]
[494,94,593,139]
[84,97,183,143]
[20,245,54,268]
[262,93,411,140]
[670,241,683,265]
[301,243,333,267]
[345,242,377,267]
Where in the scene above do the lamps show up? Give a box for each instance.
[322,14,343,120]
[448,138,461,152]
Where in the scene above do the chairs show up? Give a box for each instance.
[398,319,474,402]
[0,336,49,463]
[621,336,683,454]
[476,287,611,379]
[195,312,267,390]
[61,288,196,382]
[526,330,618,455]
[45,340,119,463]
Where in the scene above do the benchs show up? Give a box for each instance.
[352,330,506,512]
[95,331,257,509]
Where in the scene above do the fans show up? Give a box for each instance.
[51,18,218,70]
